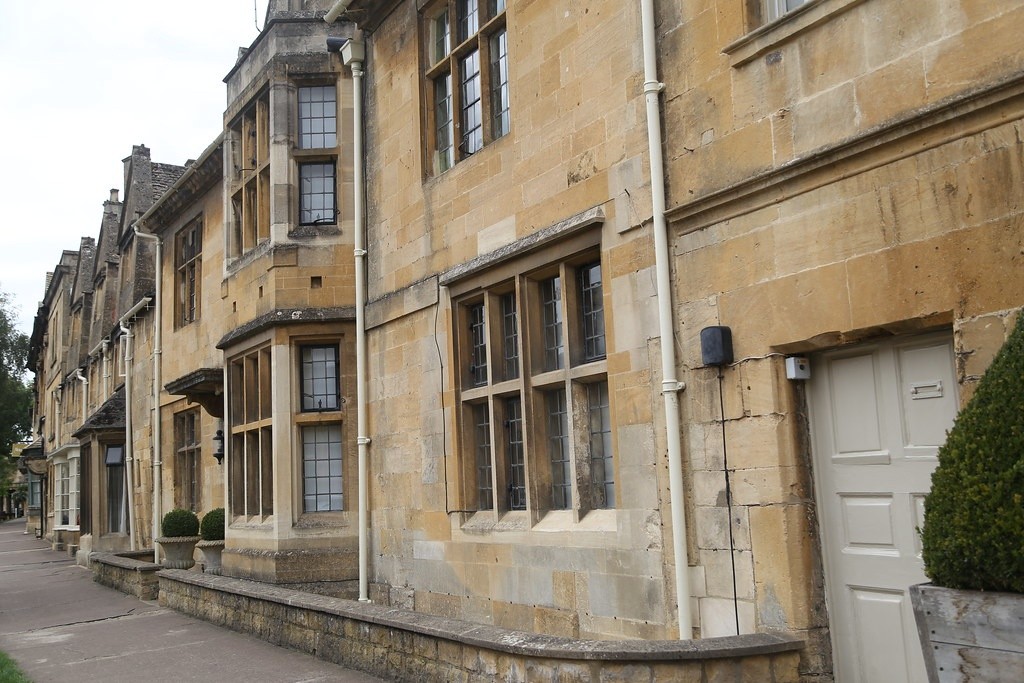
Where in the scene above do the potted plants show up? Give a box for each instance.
[907,305,1024,683]
[155,509,202,569]
[195,507,225,575]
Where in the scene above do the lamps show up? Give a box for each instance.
[212,430,224,464]
